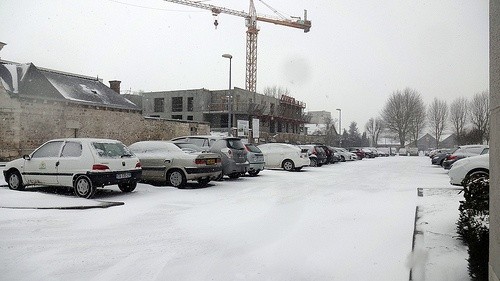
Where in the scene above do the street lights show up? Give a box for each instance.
[336,108,341,135]
[222,54,233,136]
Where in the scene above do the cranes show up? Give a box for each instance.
[163,0,312,92]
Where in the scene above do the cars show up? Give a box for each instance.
[297,144,398,167]
[170,135,250,178]
[398,148,407,156]
[425,145,489,169]
[3,137,143,199]
[448,150,489,197]
[242,143,266,177]
[408,147,420,156]
[126,141,222,188]
[255,143,311,172]
